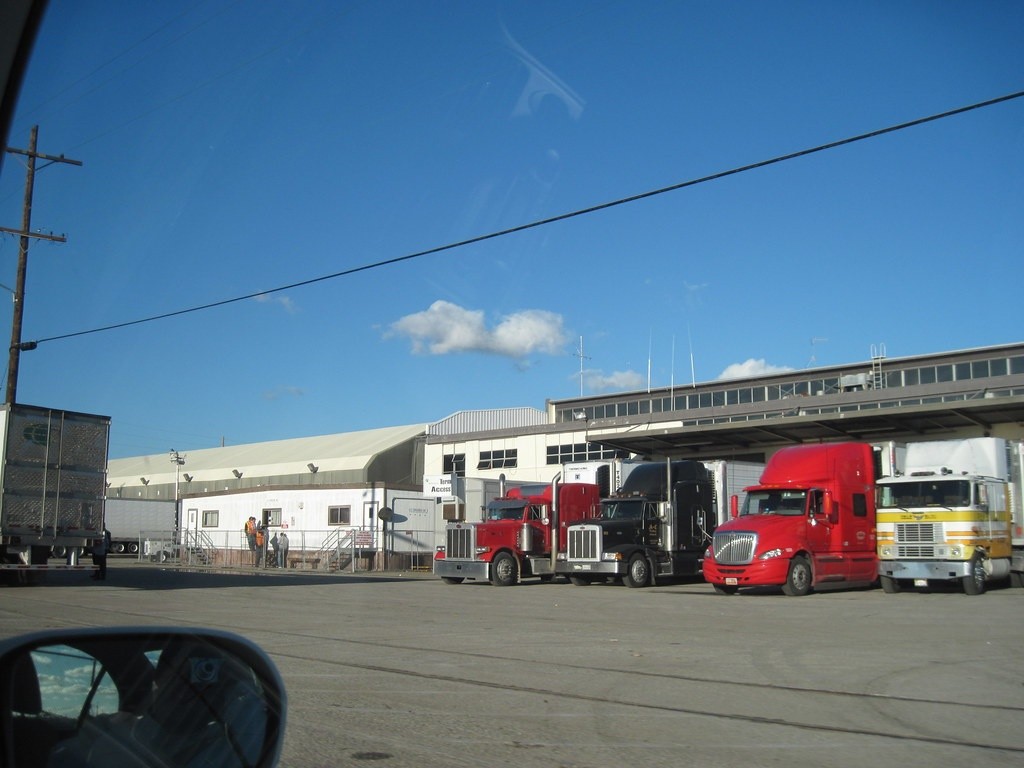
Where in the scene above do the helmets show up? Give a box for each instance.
[249,517,256,520]
[258,526,263,530]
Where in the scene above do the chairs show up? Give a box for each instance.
[10,652,60,768]
[777,501,791,509]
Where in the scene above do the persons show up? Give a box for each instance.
[243,516,289,568]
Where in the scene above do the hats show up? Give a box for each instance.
[262,524,267,527]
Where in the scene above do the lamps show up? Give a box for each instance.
[233,470,243,479]
[307,463,319,472]
[183,474,193,482]
[574,411,589,422]
[140,477,149,485]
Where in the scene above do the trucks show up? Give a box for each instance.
[553,457,770,588]
[432,458,643,587]
[703,442,875,599]
[874,434,1023,598]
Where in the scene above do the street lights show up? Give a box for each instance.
[169,448,186,545]
